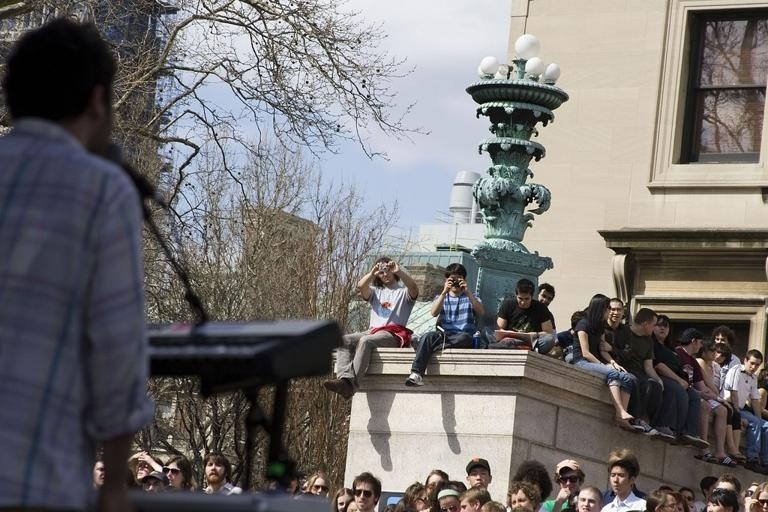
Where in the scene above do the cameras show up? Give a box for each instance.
[379,264,389,273]
[450,275,464,288]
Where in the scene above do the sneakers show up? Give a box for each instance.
[405,371,424,386]
[325,378,354,397]
[745,458,768,475]
[411,335,421,350]
[620,417,711,449]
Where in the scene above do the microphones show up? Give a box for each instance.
[97,141,168,211]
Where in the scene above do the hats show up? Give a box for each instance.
[143,470,169,486]
[466,458,490,474]
[676,328,706,344]
[554,459,580,474]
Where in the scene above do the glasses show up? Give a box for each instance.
[442,504,457,512]
[314,485,327,491]
[746,490,754,496]
[354,489,373,497]
[559,474,580,483]
[758,499,767,504]
[162,466,183,475]
[682,495,693,500]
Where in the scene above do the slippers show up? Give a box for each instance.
[718,457,738,467]
[728,452,746,460]
[695,451,719,464]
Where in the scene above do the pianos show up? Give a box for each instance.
[147,318,344,399]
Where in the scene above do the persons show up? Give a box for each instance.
[324,257,419,399]
[405,263,486,387]
[0,20,154,512]
[94,447,767,512]
[493,279,767,476]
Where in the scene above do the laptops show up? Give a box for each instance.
[494,330,538,350]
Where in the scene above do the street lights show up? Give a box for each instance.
[463,32,568,337]
